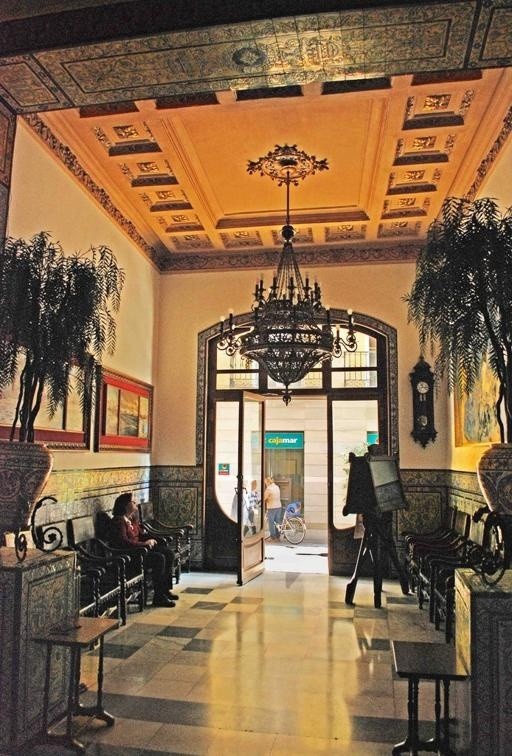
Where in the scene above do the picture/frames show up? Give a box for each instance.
[0,340,154,453]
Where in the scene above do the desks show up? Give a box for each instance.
[390,640,468,756]
[29,617,120,756]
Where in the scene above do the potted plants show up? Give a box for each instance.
[0,230,126,526]
[400,197,512,514]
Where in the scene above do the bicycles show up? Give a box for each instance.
[248,498,306,545]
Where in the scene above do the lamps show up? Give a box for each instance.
[216,144,357,406]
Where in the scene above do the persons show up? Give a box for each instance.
[264,478,282,543]
[244,479,261,538]
[107,493,179,607]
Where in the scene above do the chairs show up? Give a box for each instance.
[401,505,485,643]
[4,501,193,650]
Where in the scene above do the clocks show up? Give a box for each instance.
[409,350,438,449]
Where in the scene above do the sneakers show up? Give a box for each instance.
[153,592,178,607]
[267,534,280,543]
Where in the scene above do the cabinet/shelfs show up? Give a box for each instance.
[0,545,77,756]
[454,569,512,756]
